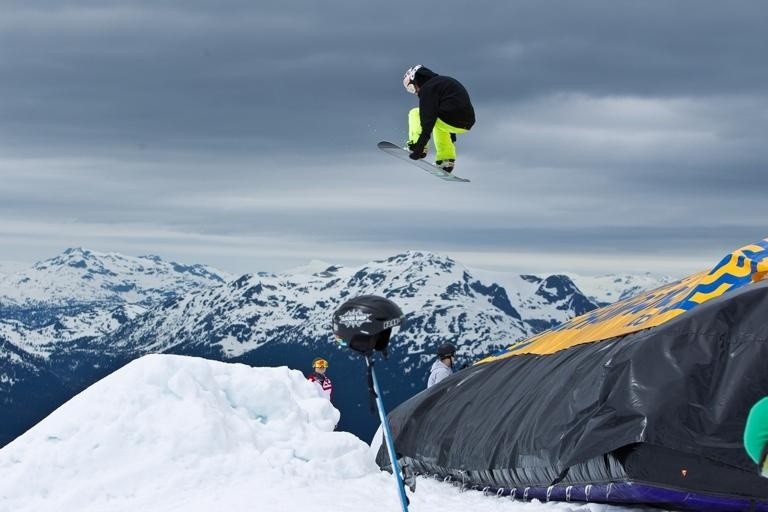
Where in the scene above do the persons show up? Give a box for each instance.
[401,62,476,173]
[424,343,458,390]
[306,357,333,405]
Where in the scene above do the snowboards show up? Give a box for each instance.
[377,141,470,182]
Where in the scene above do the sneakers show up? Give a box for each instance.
[432,159,455,173]
[402,145,427,158]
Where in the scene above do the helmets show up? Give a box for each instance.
[436,343,457,359]
[330,294,402,357]
[403,63,424,95]
[311,357,329,372]
